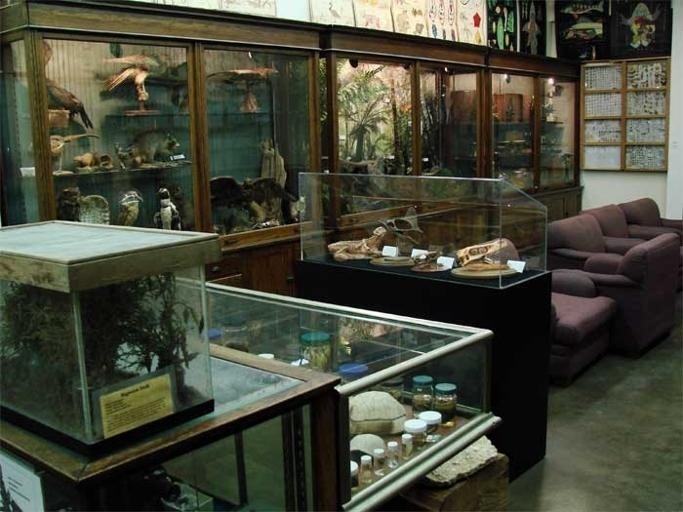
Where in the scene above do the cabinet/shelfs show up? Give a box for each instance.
[329,22,488,251]
[488,54,584,257]
[290,173,549,478]
[1,220,225,456]
[8,4,326,303]
[1,344,340,512]
[582,58,669,176]
[181,279,501,504]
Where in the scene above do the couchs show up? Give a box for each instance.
[541,214,646,272]
[560,232,682,353]
[618,195,683,236]
[534,272,621,387]
[584,204,677,256]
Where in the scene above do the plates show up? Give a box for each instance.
[411,266,444,273]
[370,260,414,267]
[451,267,517,279]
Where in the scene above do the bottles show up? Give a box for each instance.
[412,374,433,417]
[350,461,359,491]
[374,449,385,476]
[200,327,223,345]
[332,362,370,395]
[418,411,442,433]
[379,376,404,403]
[433,383,457,423]
[221,315,248,352]
[401,434,413,460]
[404,419,427,447]
[361,455,373,483]
[300,331,331,370]
[387,441,399,468]
